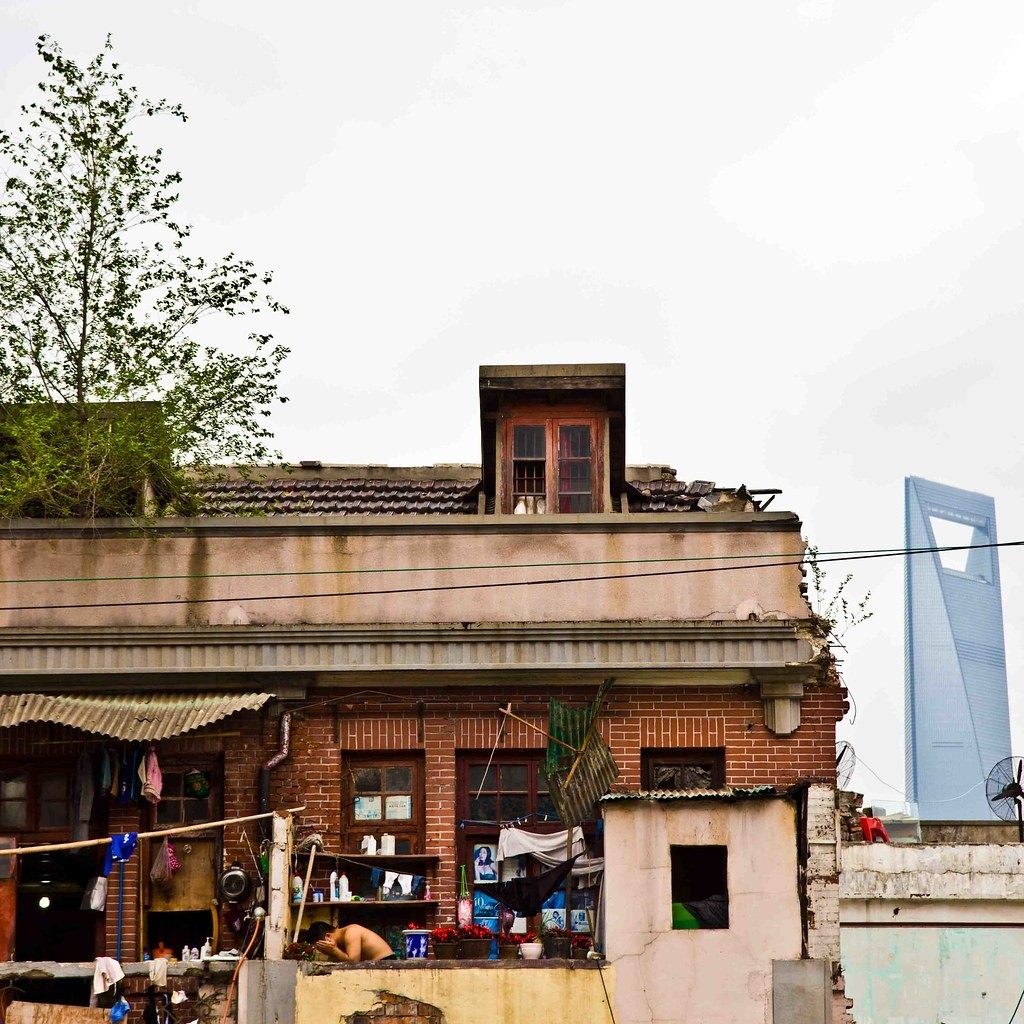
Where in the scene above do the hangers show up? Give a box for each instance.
[70,738,152,755]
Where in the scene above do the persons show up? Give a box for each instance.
[552,911,564,930]
[474,846,497,880]
[306,921,397,964]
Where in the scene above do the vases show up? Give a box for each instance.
[520,943,544,960]
[432,943,458,960]
[572,949,590,959]
[497,944,520,960]
[462,939,493,959]
[402,929,433,960]
[545,938,571,959]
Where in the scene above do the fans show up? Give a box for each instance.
[986,756,1024,843]
[835,740,856,791]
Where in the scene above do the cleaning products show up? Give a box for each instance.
[330,869,339,902]
[339,871,349,902]
[292,872,303,903]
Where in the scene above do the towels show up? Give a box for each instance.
[89,956,125,1008]
[101,744,163,804]
[495,824,605,876]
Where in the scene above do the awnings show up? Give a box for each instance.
[0,686,276,742]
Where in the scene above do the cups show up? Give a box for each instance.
[312,891,323,902]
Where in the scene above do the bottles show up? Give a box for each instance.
[339,872,348,902]
[330,870,339,901]
[380,833,396,856]
[294,873,303,902]
[181,944,190,961]
[143,946,150,960]
[361,835,376,855]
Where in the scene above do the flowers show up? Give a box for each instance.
[521,932,541,943]
[282,941,316,961]
[499,933,521,944]
[406,922,426,930]
[430,927,460,943]
[571,935,593,949]
[541,926,573,939]
[461,922,496,940]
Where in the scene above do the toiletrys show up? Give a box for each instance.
[424,880,432,900]
[143,935,214,961]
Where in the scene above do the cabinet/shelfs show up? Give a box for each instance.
[289,852,442,905]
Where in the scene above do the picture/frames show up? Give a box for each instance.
[474,844,499,883]
[542,908,567,930]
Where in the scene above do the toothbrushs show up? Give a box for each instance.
[309,883,316,893]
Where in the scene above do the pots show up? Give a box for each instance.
[218,862,251,907]
